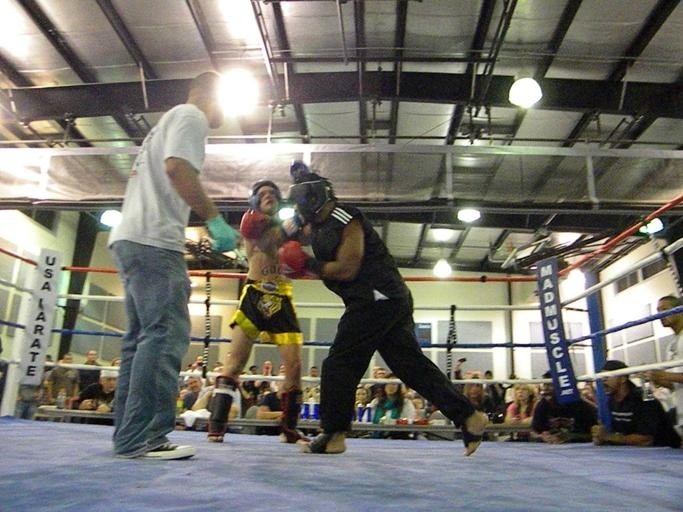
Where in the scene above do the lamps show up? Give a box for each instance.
[431,244,452,281]
[507,62,542,107]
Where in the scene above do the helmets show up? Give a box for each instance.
[139,442,194,459]
[250,180,282,208]
[290,174,335,222]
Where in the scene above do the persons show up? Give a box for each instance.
[106,71,241,462]
[72,370,120,426]
[353,388,375,425]
[532,370,596,444]
[483,370,506,410]
[193,367,243,435]
[254,361,279,401]
[412,397,429,441]
[399,383,413,400]
[48,352,81,422]
[592,359,681,449]
[188,353,204,370]
[179,375,203,431]
[250,366,261,400]
[256,374,286,436]
[463,372,504,428]
[504,383,539,443]
[581,384,595,402]
[370,375,414,440]
[278,364,288,387]
[369,367,388,396]
[429,403,458,442]
[73,351,103,398]
[282,173,490,457]
[300,367,323,402]
[642,295,683,447]
[238,372,254,417]
[207,179,316,446]
[112,357,122,367]
[15,384,50,422]
[44,355,54,371]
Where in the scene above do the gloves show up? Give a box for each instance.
[239,210,269,239]
[277,241,319,281]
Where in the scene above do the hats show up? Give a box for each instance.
[100,369,119,379]
[602,360,629,378]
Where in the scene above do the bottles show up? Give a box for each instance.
[642,378,654,400]
[58,387,65,409]
